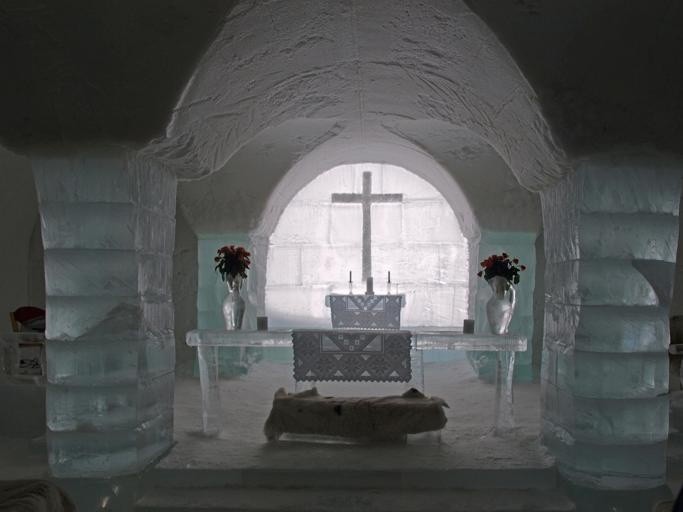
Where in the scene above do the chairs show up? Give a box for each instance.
[9,305,46,374]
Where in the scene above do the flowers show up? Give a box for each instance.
[475,252,525,282]
[214,245,251,280]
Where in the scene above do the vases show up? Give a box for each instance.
[486,276,516,335]
[222,271,245,331]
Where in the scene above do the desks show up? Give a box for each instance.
[184,329,527,440]
[324,293,405,331]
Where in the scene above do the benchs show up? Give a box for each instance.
[264,388,449,444]
